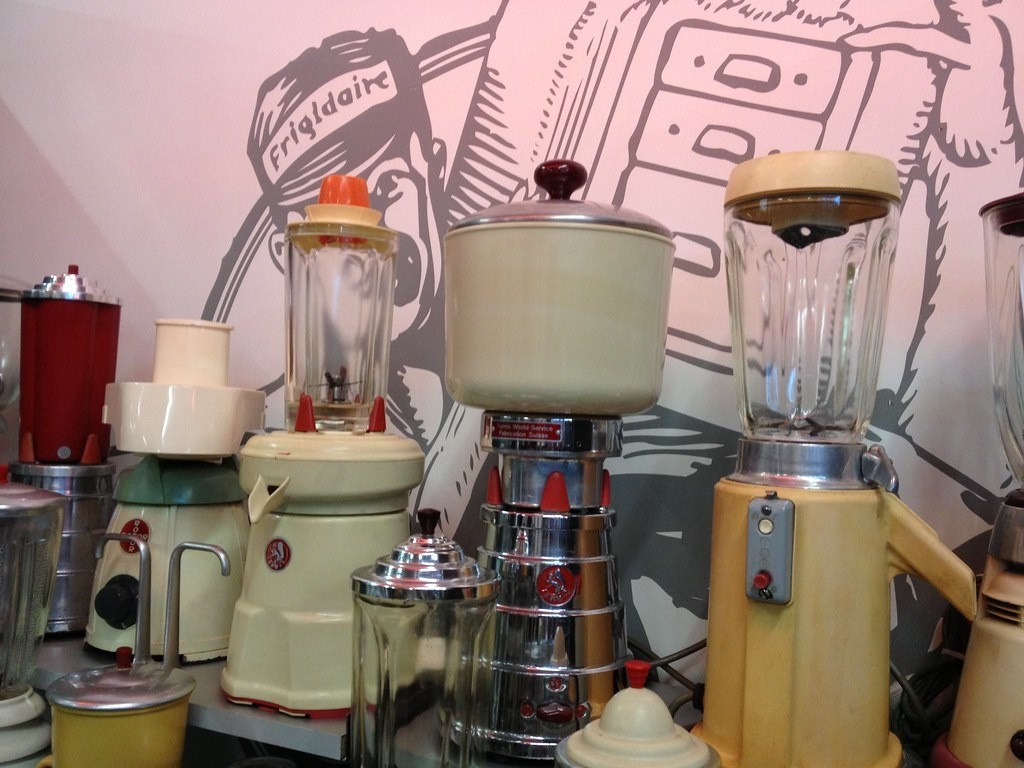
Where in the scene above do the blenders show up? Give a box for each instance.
[0,148,1024,768]
